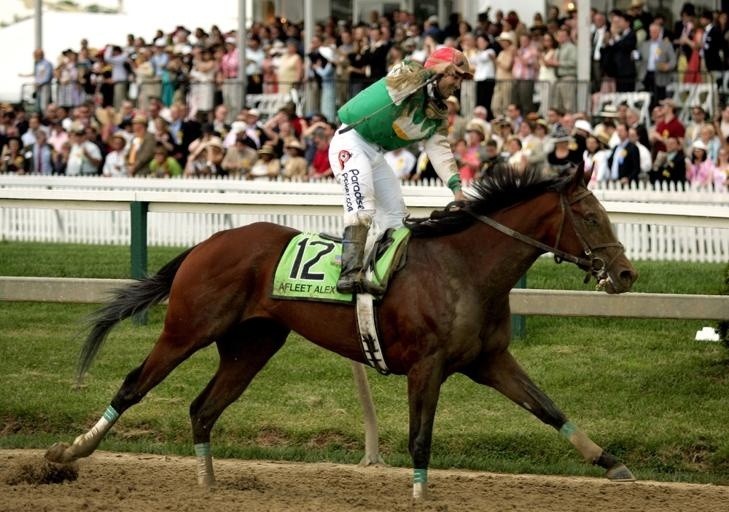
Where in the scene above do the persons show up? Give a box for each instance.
[328,47,474,294]
[2,1,729,193]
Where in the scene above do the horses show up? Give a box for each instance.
[639,324,639,325]
[44,160,640,505]
[43,335,44,337]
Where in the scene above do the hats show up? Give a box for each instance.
[201,109,306,154]
[496,32,513,44]
[465,118,610,148]
[94,107,123,127]
[446,95,460,110]
[659,98,675,107]
[693,140,707,150]
[318,44,341,62]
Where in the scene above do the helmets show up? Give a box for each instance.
[423,47,474,80]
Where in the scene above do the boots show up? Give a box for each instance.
[336,224,386,297]
